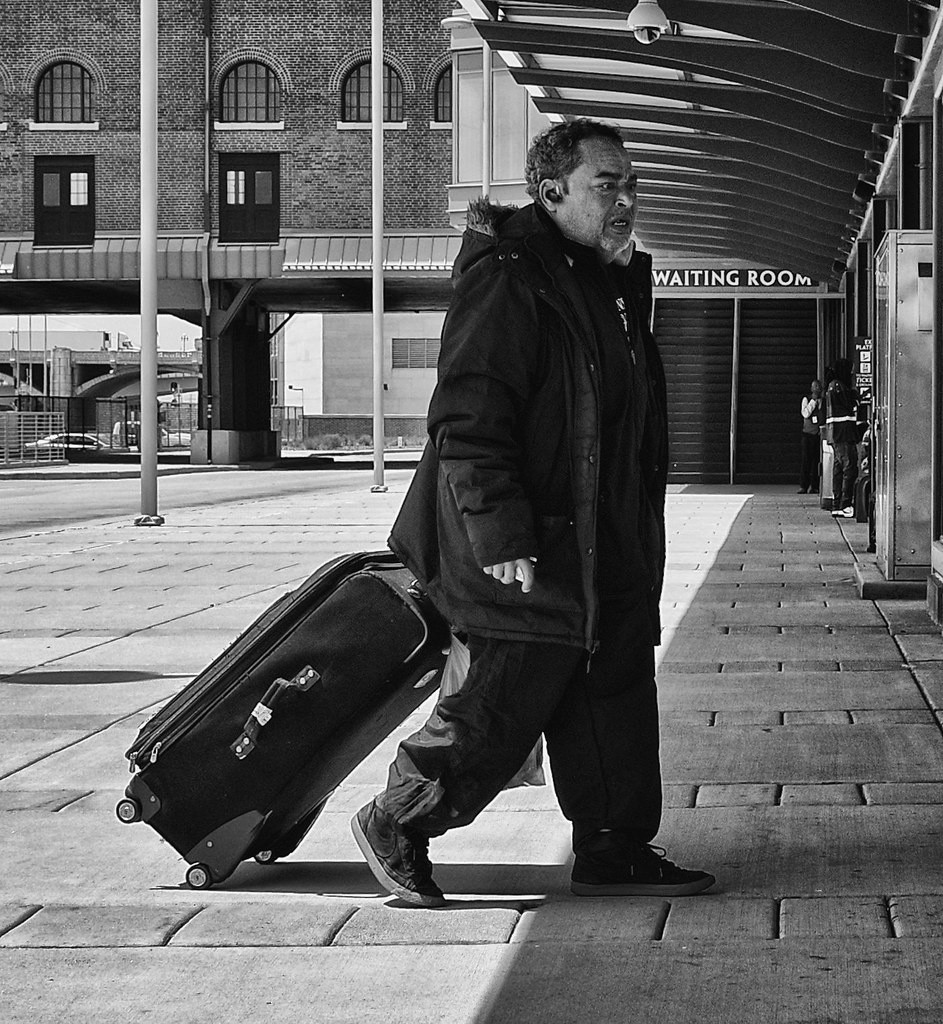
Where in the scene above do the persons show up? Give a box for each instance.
[827,358,871,518]
[350,118,717,906]
[797,380,826,494]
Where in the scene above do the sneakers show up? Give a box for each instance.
[570,844,717,898]
[351,797,449,909]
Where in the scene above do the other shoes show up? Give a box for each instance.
[832,505,856,519]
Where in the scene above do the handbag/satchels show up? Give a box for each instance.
[436,624,546,788]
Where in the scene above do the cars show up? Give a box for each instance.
[19,417,193,456]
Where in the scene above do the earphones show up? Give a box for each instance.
[546,189,561,202]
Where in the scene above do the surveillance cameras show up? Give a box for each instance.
[627,3,670,45]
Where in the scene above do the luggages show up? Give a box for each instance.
[117,548,453,889]
